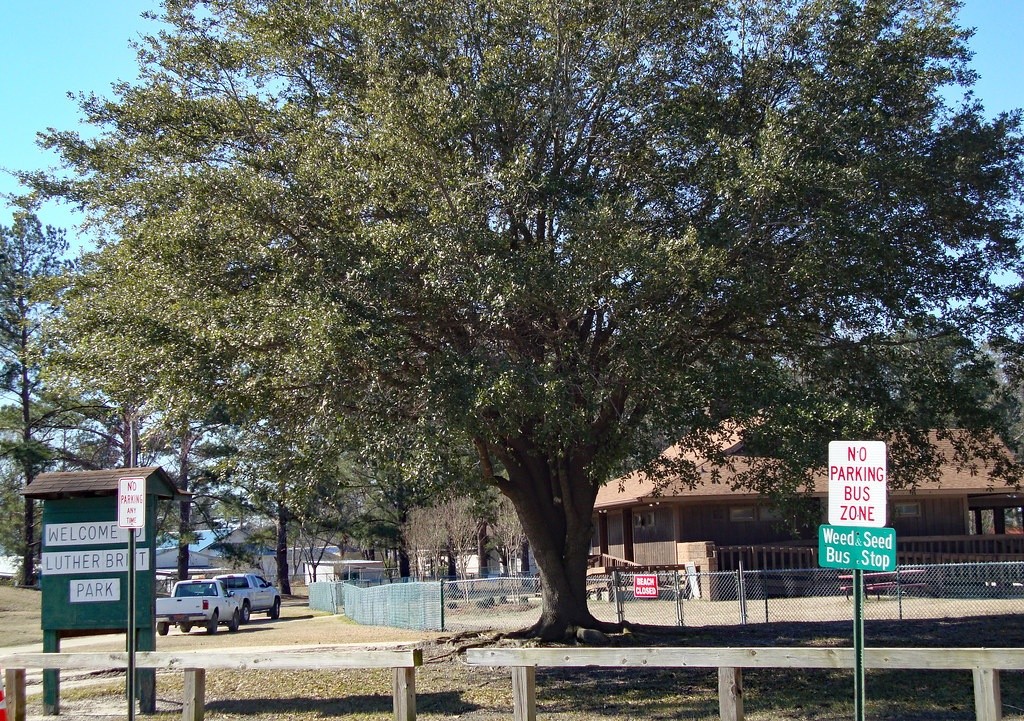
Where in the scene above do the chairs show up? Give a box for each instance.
[205,588,215,595]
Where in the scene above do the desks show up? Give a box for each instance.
[837,568,925,599]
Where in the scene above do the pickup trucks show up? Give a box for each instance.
[156,578,241,636]
[195,573,281,624]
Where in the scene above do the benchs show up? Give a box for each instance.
[840,581,927,600]
